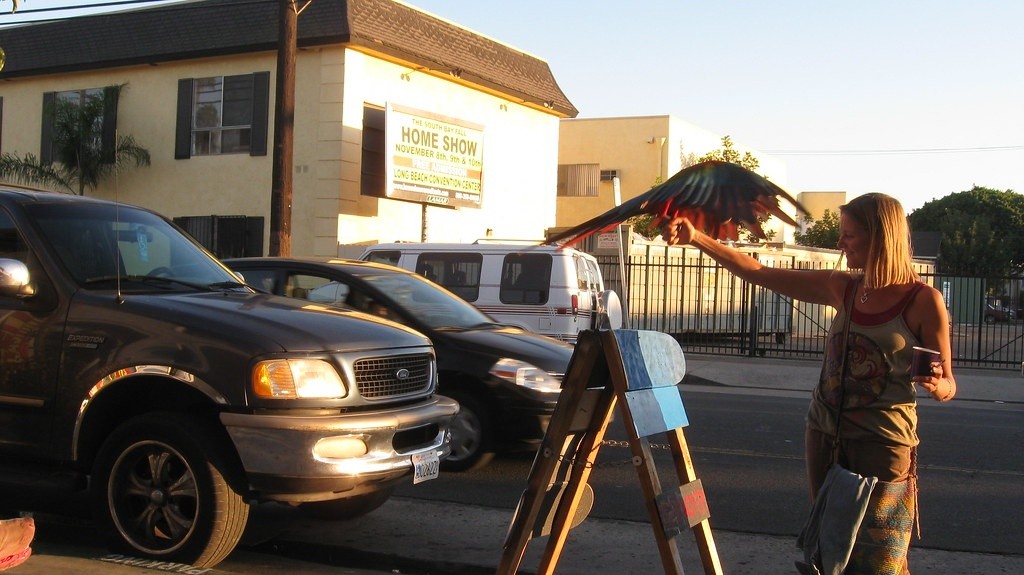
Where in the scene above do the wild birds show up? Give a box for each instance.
[535,159,811,252]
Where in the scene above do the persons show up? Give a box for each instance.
[660,192,957,575]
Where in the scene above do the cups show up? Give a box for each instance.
[910,345,940,388]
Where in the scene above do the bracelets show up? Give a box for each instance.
[938,378,952,402]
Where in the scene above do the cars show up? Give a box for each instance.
[984,299,1016,324]
[152,254,579,473]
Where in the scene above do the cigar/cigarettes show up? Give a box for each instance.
[936,360,945,368]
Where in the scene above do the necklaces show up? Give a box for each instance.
[860,283,879,303]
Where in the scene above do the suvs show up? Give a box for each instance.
[306,242,623,349]
[0,184,461,572]
